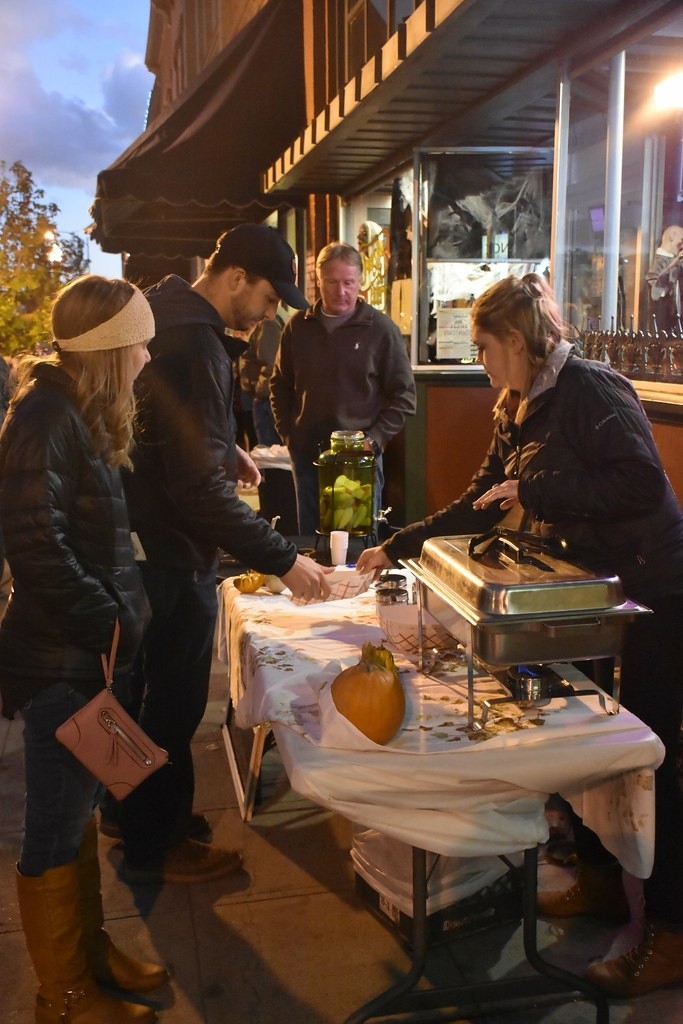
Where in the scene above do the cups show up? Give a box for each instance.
[330,531,348,564]
[375,589,408,604]
[375,574,408,590]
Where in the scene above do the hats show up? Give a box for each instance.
[218,224,310,310]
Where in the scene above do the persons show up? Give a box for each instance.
[357,219,386,315]
[650,237,683,333]
[221,313,287,492]
[0,341,56,620]
[99,220,336,883]
[350,272,683,999]
[0,275,177,1024]
[644,225,683,331]
[268,242,417,538]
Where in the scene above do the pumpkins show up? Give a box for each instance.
[331,665,405,745]
[359,643,400,678]
[236,572,261,593]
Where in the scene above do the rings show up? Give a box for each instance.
[492,483,500,490]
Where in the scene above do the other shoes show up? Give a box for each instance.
[99,818,123,839]
[127,839,245,885]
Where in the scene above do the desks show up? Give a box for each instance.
[216,570,667,1024]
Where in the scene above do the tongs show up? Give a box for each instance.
[467,526,567,559]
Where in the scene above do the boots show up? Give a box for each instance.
[540,857,631,917]
[16,859,156,1024]
[585,919,683,998]
[75,818,166,989]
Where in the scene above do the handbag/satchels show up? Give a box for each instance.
[55,618,170,800]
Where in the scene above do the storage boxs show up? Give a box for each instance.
[350,828,524,942]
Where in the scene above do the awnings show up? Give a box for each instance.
[82,0,308,261]
